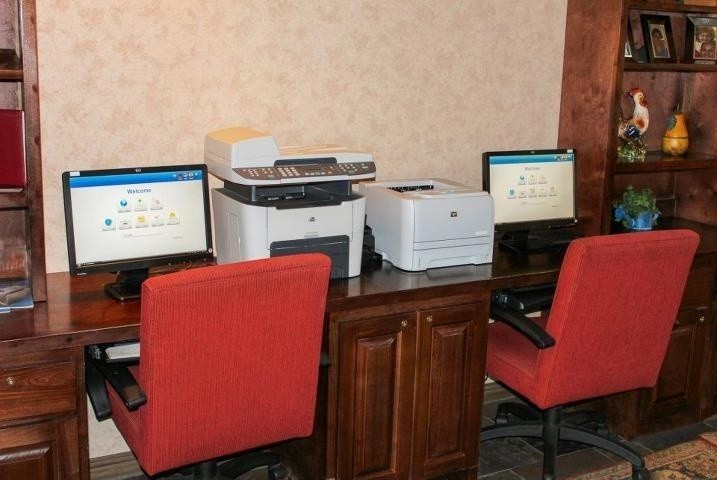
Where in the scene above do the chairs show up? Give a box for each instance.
[488,230,700,480]
[83,253,332,480]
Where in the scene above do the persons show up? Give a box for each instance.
[652,27,664,54]
[700,39,714,59]
[692,24,713,54]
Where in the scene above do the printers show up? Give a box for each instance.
[359,178,495,271]
[204,127,377,279]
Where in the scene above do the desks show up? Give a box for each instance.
[0,239,559,480]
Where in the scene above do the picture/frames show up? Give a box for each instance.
[639,11,678,64]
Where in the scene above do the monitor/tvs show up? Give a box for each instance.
[61,163,213,301]
[482,148,578,255]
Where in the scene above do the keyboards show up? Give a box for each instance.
[106,343,140,360]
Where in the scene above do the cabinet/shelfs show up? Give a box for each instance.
[0,0,49,304]
[557,0,717,439]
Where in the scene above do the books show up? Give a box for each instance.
[0,108,36,315]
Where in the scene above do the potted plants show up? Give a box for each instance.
[617,185,659,231]
